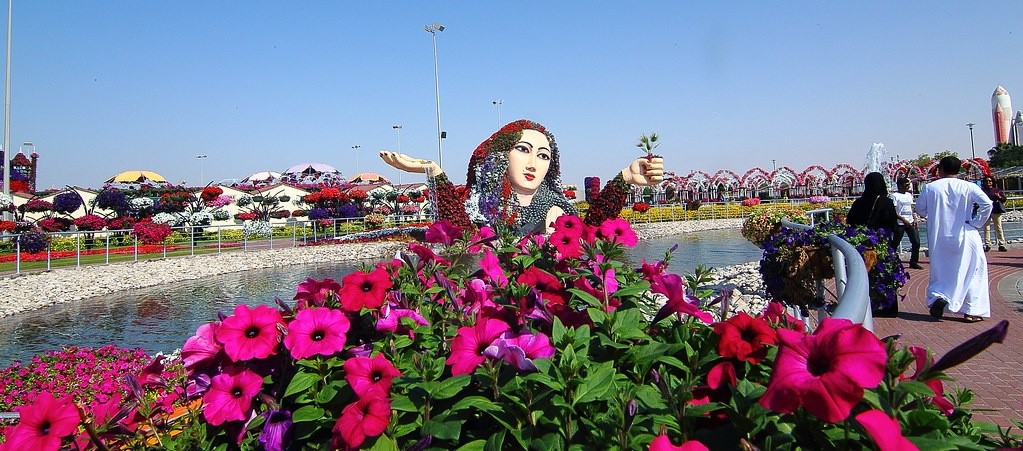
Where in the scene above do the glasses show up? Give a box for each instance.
[986,180,992,182]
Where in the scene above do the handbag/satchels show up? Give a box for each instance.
[994,201,1006,213]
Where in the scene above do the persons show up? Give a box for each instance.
[847,156,1009,322]
[379,120,665,238]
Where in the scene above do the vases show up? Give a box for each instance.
[861,246,878,277]
[801,242,832,283]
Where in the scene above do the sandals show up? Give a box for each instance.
[963,315,983,322]
[930,299,947,318]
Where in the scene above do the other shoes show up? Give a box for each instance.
[909,264,921,269]
[999,246,1007,252]
[985,246,991,252]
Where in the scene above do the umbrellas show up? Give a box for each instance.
[104,170,168,183]
[242,161,389,184]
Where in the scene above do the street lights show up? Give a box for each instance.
[422,22,447,171]
[493,100,503,129]
[965,122,977,180]
[392,125,404,188]
[352,144,361,175]
[196,154,207,186]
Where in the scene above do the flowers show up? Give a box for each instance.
[0,118,1023,451]
